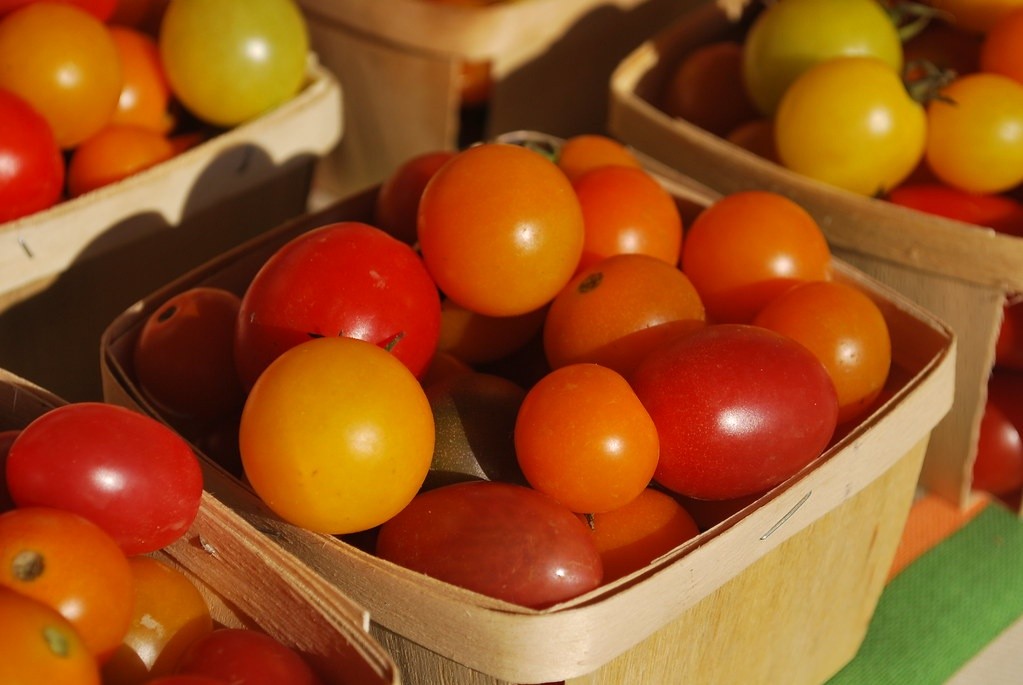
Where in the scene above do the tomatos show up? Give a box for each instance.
[0,0,1023,685]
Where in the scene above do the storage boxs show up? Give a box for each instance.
[100,130,959,685]
[0,66,345,402]
[0,367,403,685]
[292,0,699,199]
[607,0,1023,515]
